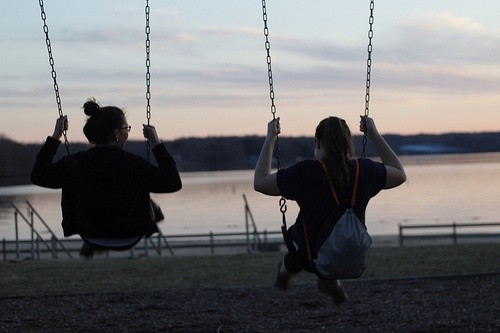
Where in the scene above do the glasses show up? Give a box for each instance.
[117,126,131,132]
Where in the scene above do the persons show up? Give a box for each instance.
[29,99,183,260]
[254,116,407,307]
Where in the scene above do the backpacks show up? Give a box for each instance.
[299,156,373,281]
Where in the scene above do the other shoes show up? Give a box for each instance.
[274,263,288,290]
[333,289,349,305]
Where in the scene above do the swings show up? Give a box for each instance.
[39,0,150,252]
[262,0,373,280]
